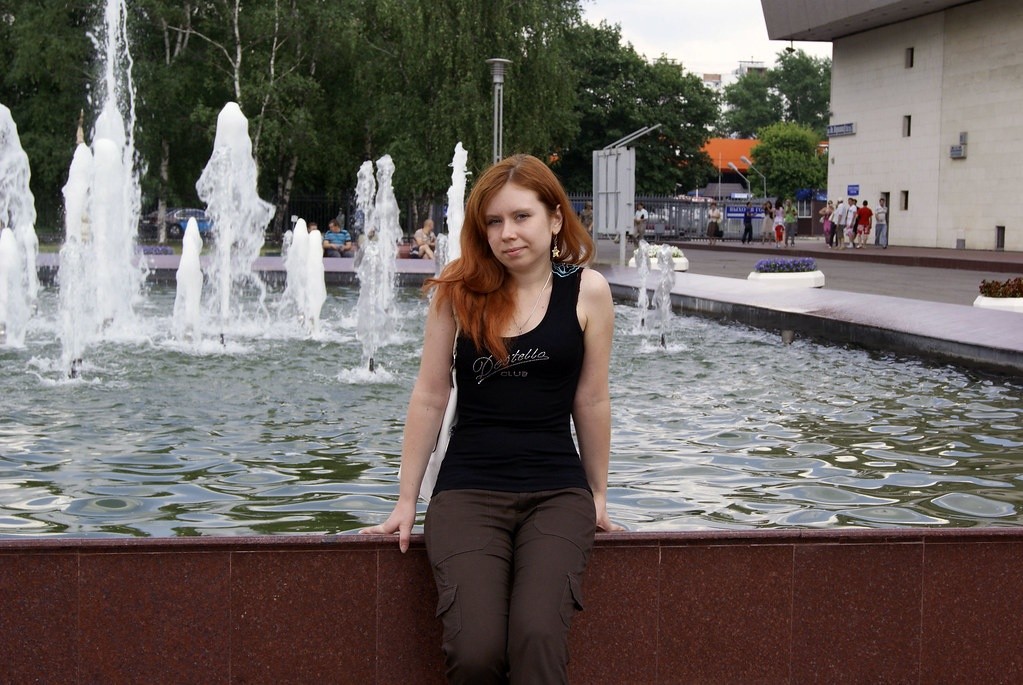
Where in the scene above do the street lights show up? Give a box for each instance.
[727,162,750,201]
[741,156,766,200]
[484,58,513,167]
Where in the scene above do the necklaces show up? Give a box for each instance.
[510,272,552,335]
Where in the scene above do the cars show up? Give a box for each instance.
[645,194,718,239]
[139,208,211,238]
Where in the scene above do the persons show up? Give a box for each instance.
[741,202,755,244]
[359,153,624,685]
[706,203,723,246]
[307,222,324,255]
[322,219,354,257]
[760,199,798,247]
[819,198,873,249]
[408,218,436,259]
[874,198,888,249]
[634,203,649,241]
[578,201,593,234]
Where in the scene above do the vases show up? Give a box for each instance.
[628,256,689,272]
[747,270,826,288]
[973,295,1022,312]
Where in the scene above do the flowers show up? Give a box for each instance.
[979,275,1023,295]
[755,257,816,270]
[631,243,684,257]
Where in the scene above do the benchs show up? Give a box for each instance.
[348,236,416,259]
